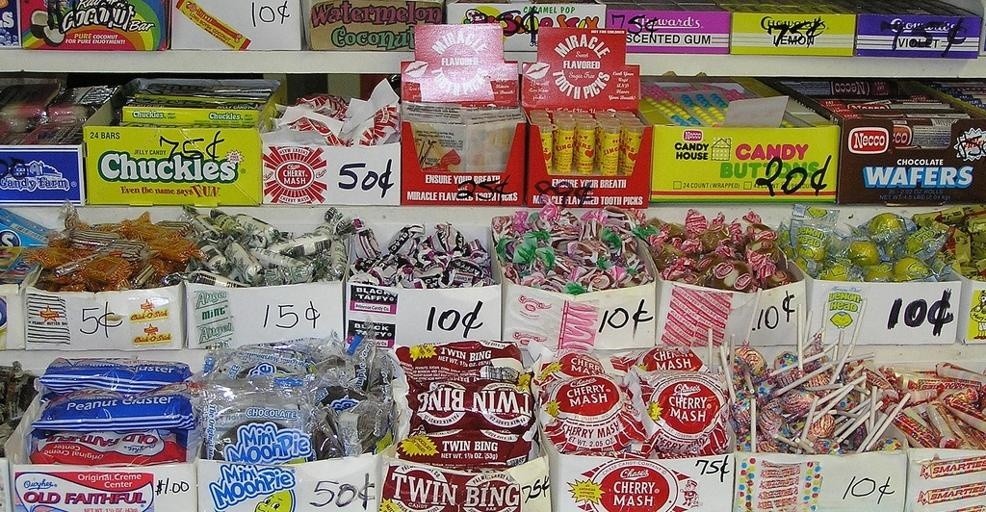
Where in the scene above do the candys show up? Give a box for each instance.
[0,204,985,512]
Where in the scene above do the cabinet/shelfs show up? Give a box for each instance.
[1,49,986,377]
